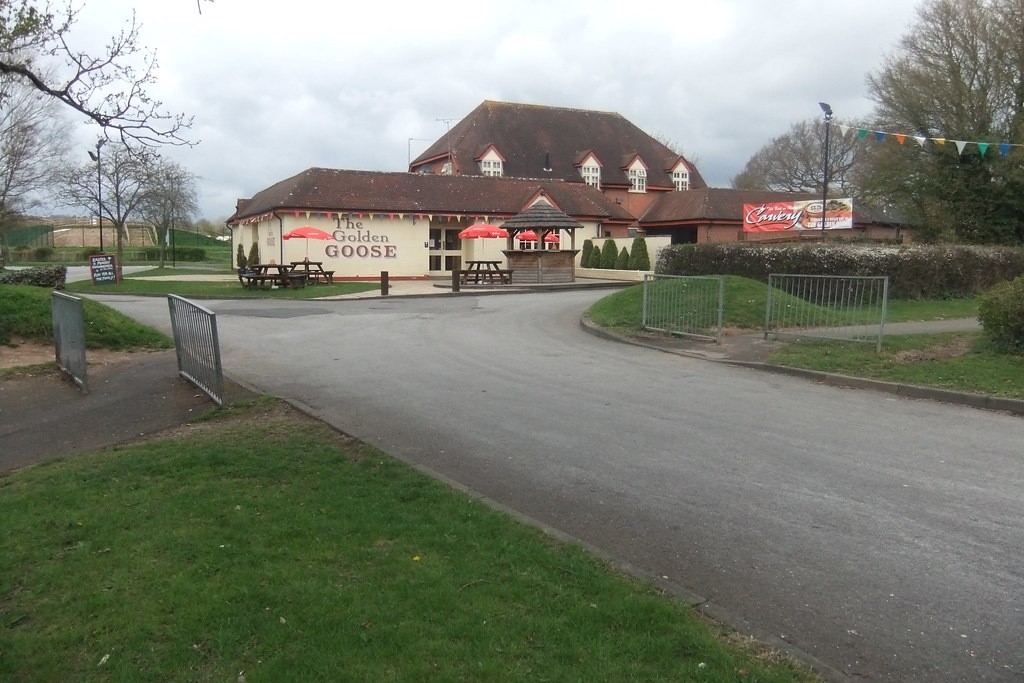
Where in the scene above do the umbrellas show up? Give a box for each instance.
[283,224,333,258]
[459,222,559,260]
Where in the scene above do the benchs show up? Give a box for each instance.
[238,269,336,288]
[458,269,514,284]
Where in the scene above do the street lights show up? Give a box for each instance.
[818,102,832,235]
[96,139,105,255]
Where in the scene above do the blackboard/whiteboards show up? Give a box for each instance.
[89,254,117,285]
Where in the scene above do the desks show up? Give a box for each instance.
[461,261,509,285]
[250,264,294,288]
[287,261,329,285]
